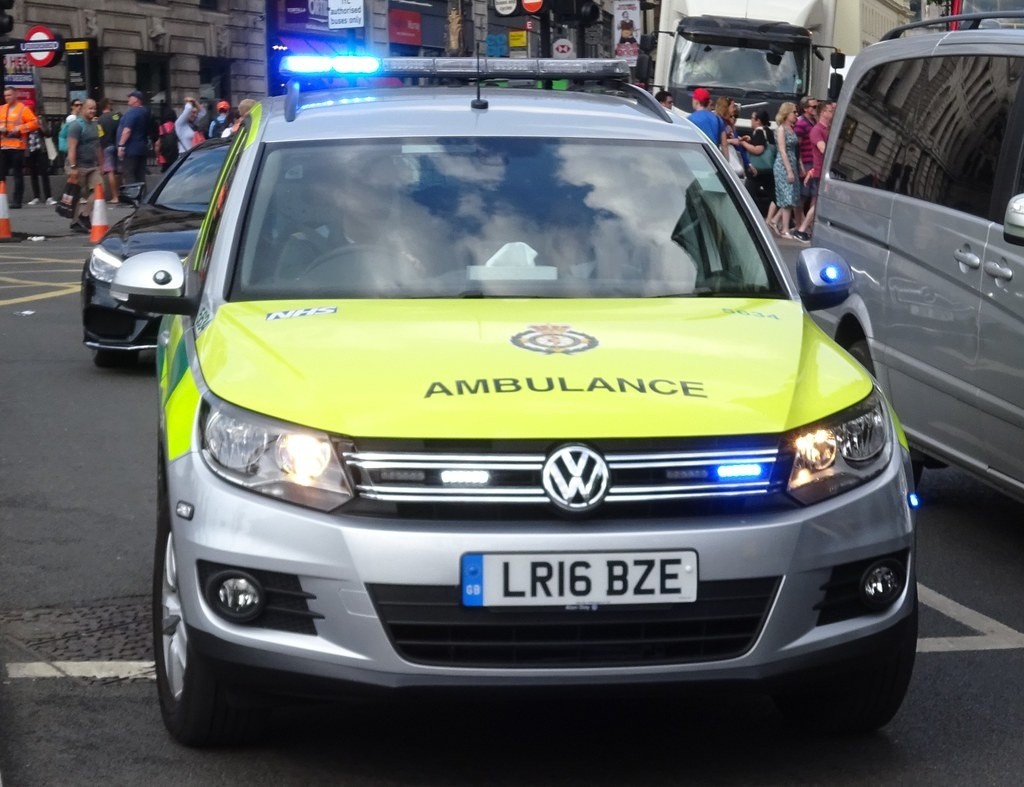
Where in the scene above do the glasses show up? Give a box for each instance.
[73,104,83,107]
[791,111,798,114]
[666,101,673,104]
[808,105,818,109]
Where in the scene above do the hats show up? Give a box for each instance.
[217,101,230,112]
[238,99,255,115]
[688,88,711,104]
[127,90,144,99]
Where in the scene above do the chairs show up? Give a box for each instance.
[271,158,410,282]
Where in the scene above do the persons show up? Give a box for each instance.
[115,91,161,207]
[617,11,638,44]
[208,101,234,138]
[92,98,123,204]
[158,109,178,173]
[0,86,58,209]
[174,96,207,157]
[655,91,674,110]
[686,88,777,226]
[268,168,427,279]
[65,99,83,123]
[766,96,837,243]
[221,99,257,138]
[64,99,106,233]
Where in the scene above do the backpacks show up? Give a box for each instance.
[58,118,86,153]
[160,124,179,163]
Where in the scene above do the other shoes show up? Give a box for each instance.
[9,203,22,208]
[70,220,89,234]
[79,197,88,204]
[768,220,780,235]
[780,231,793,239]
[78,212,92,229]
[106,200,119,204]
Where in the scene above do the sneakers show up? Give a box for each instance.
[45,197,57,205]
[27,198,39,205]
[793,229,811,243]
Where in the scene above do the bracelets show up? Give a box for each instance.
[97,162,103,166]
[70,164,77,169]
[118,145,125,147]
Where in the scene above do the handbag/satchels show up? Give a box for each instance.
[192,130,206,147]
[54,178,82,218]
[719,144,745,180]
[44,137,57,163]
[746,126,776,176]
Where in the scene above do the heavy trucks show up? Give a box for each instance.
[636,15,847,216]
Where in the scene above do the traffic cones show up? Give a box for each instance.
[0,180,22,243]
[83,182,110,246]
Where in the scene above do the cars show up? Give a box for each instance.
[82,138,235,369]
[801,11,1024,505]
[107,54,921,747]
